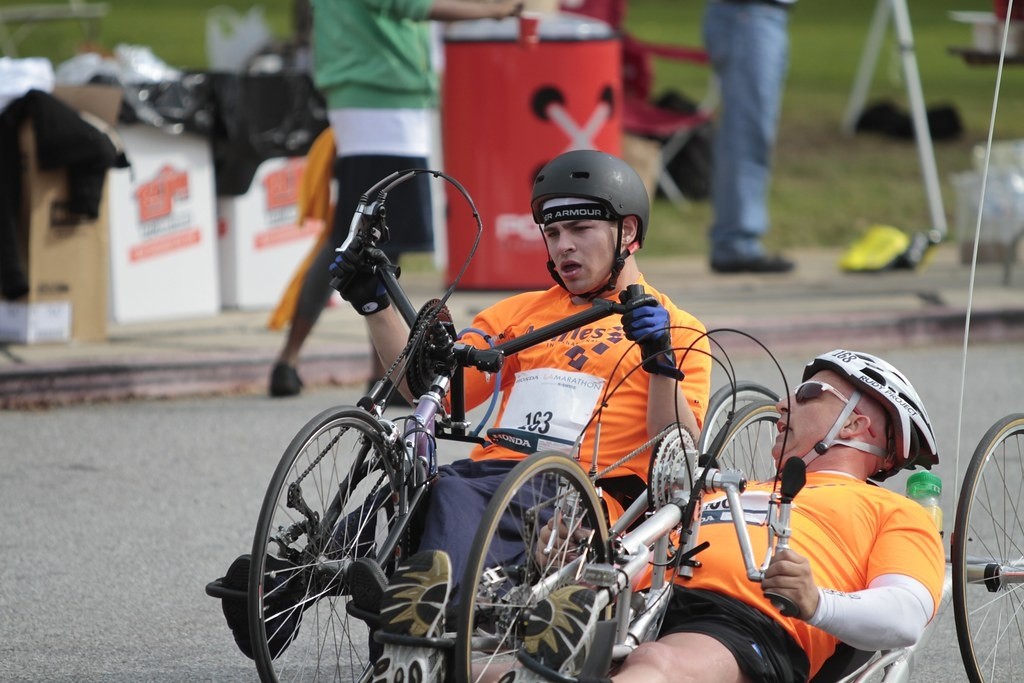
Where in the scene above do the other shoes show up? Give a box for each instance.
[221,554,294,659]
[713,250,795,273]
[347,558,388,612]
[269,360,303,396]
[367,376,403,405]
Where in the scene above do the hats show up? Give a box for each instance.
[539,199,615,224]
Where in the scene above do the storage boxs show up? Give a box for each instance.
[0,70,338,343]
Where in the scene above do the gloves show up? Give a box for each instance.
[621,296,686,380]
[331,249,391,315]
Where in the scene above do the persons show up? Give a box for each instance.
[371,349,946,683]
[268,0,524,397]
[702,0,796,273]
[222,150,712,663]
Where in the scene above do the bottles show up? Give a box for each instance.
[905,471,944,538]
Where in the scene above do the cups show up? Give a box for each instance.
[521,12,539,49]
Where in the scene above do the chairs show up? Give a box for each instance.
[560,0,724,213]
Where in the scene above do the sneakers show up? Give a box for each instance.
[513,585,599,683]
[372,549,452,683]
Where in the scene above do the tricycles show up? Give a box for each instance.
[201,167,1024,683]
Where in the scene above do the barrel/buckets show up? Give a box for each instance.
[443,22,625,292]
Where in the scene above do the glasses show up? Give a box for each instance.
[794,381,877,437]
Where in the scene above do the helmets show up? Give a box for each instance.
[802,349,940,480]
[531,150,650,249]
